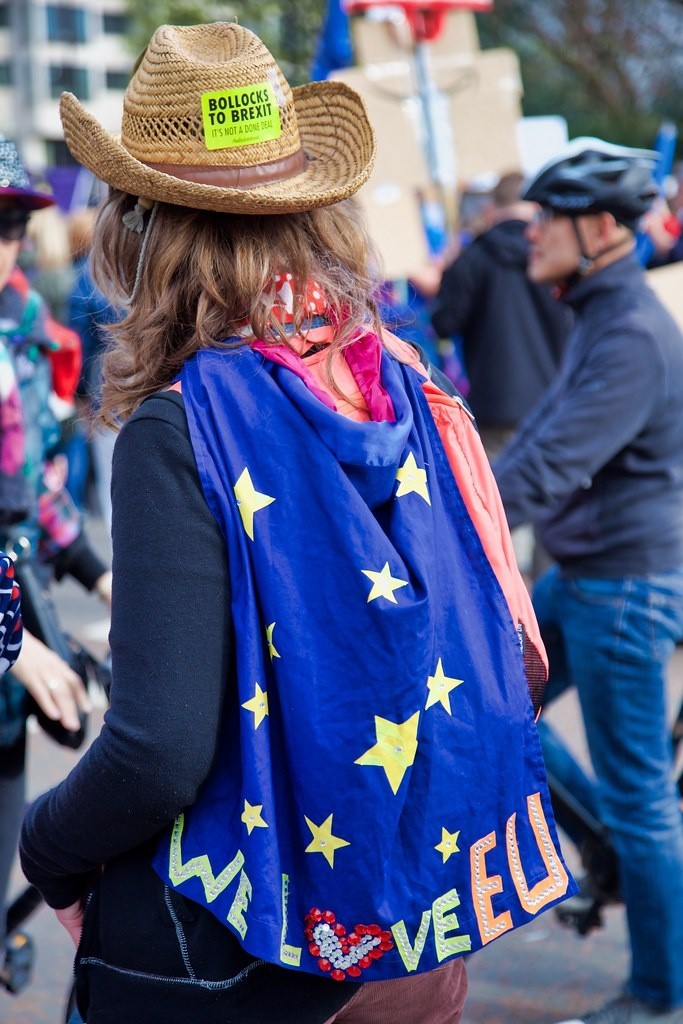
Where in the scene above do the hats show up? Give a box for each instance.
[0,134,54,210]
[60,22,377,214]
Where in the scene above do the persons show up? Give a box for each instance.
[0,136,683,1024]
[17,19,548,1024]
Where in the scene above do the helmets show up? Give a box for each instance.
[520,137,662,227]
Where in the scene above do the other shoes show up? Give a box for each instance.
[553,861,625,934]
[559,992,683,1024]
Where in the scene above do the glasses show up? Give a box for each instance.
[532,208,592,229]
[0,208,30,240]
